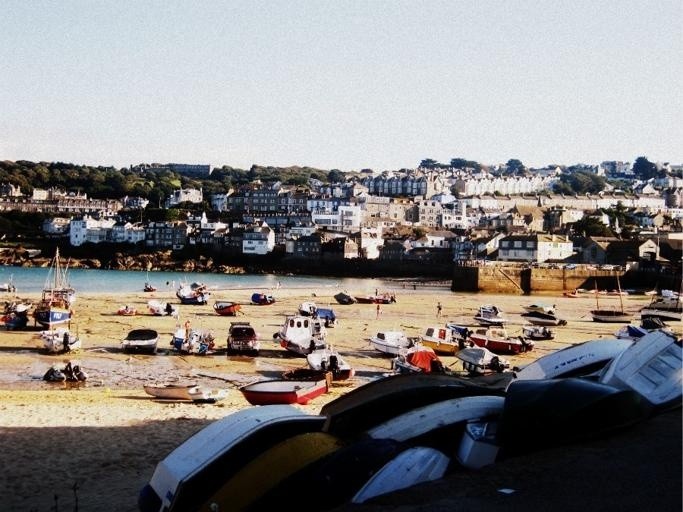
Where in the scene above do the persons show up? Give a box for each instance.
[374,303,383,321]
[434,300,443,320]
[173,320,183,355]
[374,286,378,297]
[61,330,72,352]
[183,318,192,343]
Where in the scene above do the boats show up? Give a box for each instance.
[121,328,159,354]
[44,363,88,382]
[3,304,29,331]
[37,325,81,353]
[0,274,18,293]
[123,306,136,316]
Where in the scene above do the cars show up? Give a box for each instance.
[464,259,625,271]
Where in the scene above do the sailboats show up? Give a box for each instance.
[31,246,74,330]
